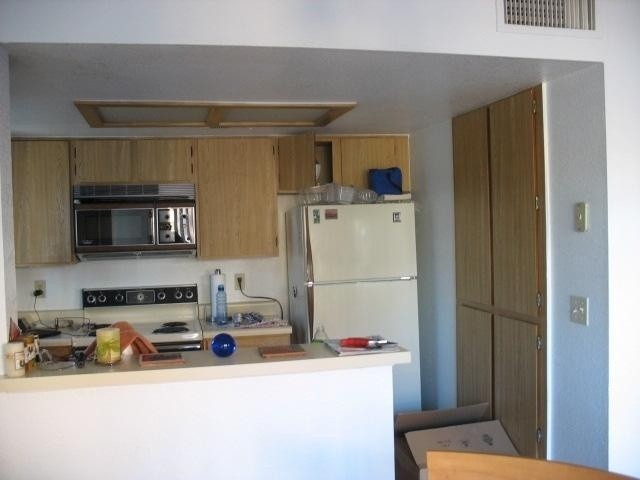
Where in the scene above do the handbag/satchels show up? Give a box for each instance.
[368,167,403,195]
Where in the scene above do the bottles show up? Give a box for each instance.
[74,346,86,369]
[209,267,227,326]
[94,327,120,367]
[4,334,41,377]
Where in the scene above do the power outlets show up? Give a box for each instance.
[234,273,246,290]
[33,279,47,298]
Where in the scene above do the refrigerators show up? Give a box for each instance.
[285,199,423,412]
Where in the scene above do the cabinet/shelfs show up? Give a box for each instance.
[452,80,548,460]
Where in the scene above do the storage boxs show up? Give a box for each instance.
[394,402,522,480]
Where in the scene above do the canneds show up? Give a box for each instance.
[6,333,39,377]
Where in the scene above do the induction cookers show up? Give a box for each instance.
[75,320,203,337]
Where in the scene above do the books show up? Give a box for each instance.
[256,343,308,358]
[136,351,185,368]
[379,191,412,202]
[324,334,401,357]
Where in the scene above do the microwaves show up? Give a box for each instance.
[73,206,196,248]
